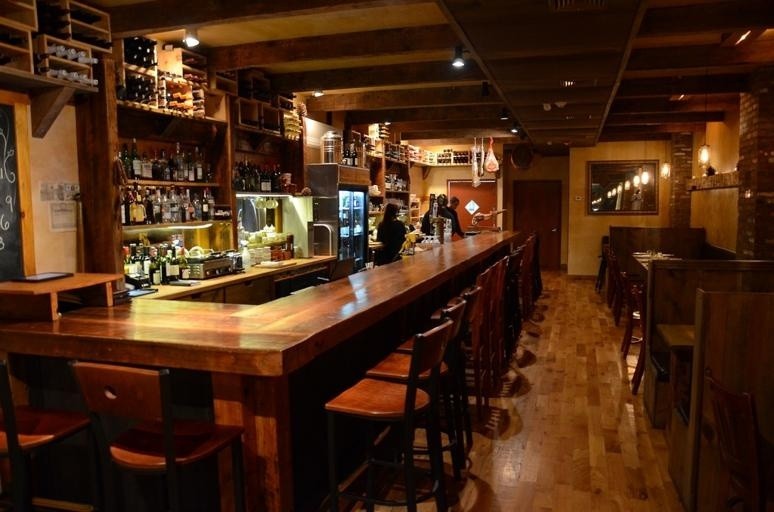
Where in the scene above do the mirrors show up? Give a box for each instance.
[588,160,660,216]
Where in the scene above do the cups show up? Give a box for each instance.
[646,249,663,262]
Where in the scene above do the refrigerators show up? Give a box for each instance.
[308,164,370,281]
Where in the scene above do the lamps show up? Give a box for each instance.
[660,117,672,179]
[452,46,521,133]
[697,67,712,167]
[607,166,652,198]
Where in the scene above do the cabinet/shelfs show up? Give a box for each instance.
[352,121,489,233]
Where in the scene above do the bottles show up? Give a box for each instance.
[115,32,232,290]
[237,220,294,267]
[235,154,297,193]
[320,130,358,165]
[366,126,486,166]
[241,76,304,135]
[371,172,452,256]
[1,2,113,88]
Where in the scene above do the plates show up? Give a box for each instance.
[510,144,533,169]
[465,231,480,234]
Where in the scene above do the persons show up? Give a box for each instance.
[422,195,448,238]
[373,202,407,267]
[447,196,465,237]
